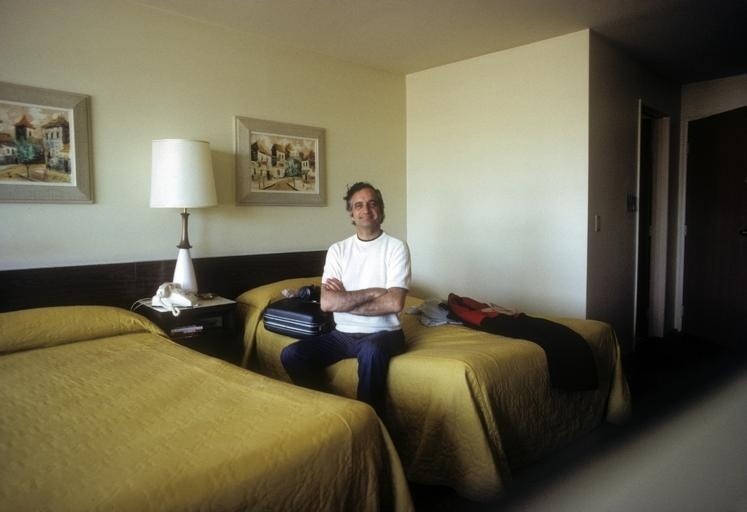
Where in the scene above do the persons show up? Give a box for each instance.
[281,182,412,420]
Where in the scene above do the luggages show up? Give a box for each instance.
[263,297,334,339]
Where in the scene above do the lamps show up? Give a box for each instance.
[149,138,218,295]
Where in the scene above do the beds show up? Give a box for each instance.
[0,333,416,512]
[253,295,632,505]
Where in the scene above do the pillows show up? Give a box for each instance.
[0,305,170,353]
[236,276,321,312]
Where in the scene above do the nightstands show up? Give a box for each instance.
[131,293,239,361]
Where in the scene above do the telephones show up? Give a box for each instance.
[151,282,199,318]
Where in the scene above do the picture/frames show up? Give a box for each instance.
[233,114,329,208]
[0,82,95,204]
